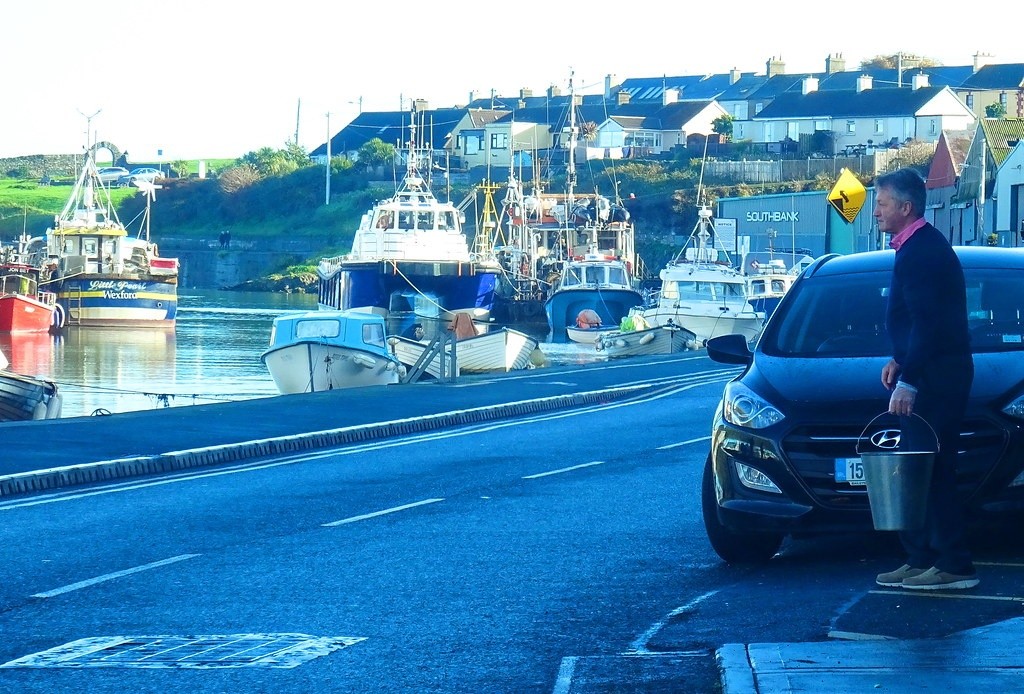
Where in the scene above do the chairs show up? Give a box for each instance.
[817,317,1023,352]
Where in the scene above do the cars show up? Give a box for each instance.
[118,167,166,187]
[700,244,1024,567]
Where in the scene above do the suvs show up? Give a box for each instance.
[94,166,129,186]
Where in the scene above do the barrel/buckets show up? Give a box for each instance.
[859,410,943,533]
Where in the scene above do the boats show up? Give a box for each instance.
[634,184,766,342]
[260,311,401,393]
[314,91,645,344]
[0,264,56,338]
[413,326,540,375]
[745,259,799,325]
[604,318,697,358]
[367,334,459,384]
[0,372,63,422]
[22,111,182,325]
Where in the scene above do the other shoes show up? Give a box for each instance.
[905,567,981,591]
[876,563,929,588]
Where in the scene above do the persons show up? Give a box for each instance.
[876,169,982,591]
